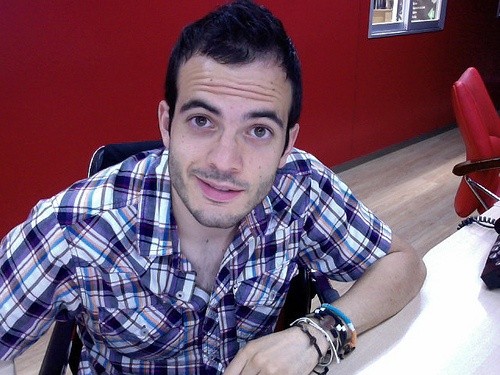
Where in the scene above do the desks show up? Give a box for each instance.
[310,201,500,375]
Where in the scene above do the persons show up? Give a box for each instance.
[0,0,430,375]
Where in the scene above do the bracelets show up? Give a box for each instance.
[290,307,348,375]
[321,302,357,352]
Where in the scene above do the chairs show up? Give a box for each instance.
[39,140,341,375]
[451,67,500,218]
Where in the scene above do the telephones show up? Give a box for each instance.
[479,216,500,288]
